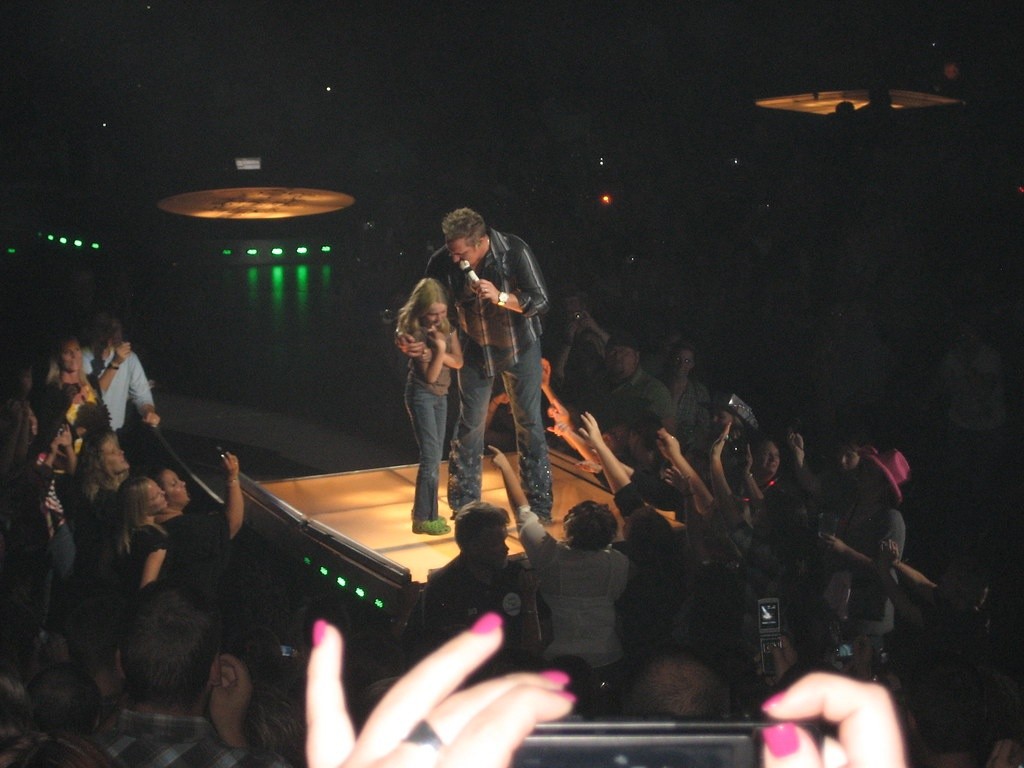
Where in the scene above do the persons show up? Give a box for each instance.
[0,293,1024,768]
[396,208,555,521]
[396,278,464,534]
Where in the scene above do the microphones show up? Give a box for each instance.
[459,260,479,282]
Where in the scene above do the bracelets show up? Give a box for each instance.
[225,477,239,484]
[108,363,119,370]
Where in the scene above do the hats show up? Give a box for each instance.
[699,392,757,429]
[857,445,911,507]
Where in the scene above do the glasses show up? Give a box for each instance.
[674,357,693,366]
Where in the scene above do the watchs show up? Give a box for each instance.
[497,291,510,306]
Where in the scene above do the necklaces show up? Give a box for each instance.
[844,500,859,531]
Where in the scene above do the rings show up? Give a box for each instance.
[723,435,730,441]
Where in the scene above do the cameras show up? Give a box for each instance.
[281,644,295,656]
[58,429,63,437]
[216,446,225,458]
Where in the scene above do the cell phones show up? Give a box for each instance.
[506,722,825,768]
[758,597,783,677]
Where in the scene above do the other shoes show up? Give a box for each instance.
[412,516,451,536]
